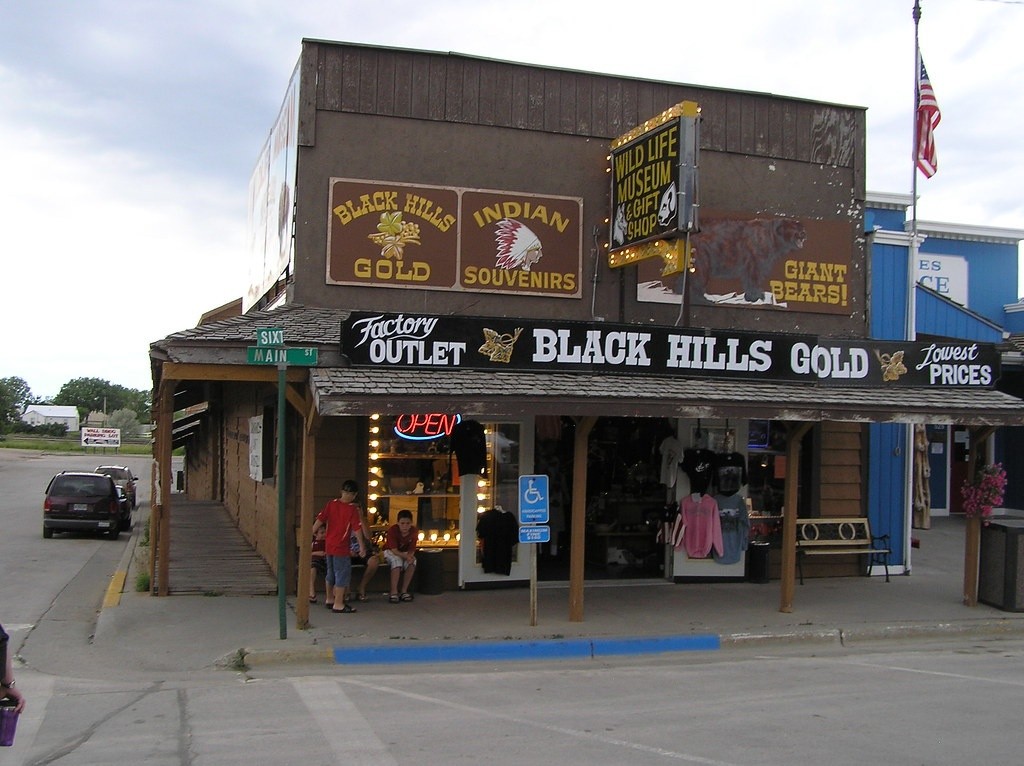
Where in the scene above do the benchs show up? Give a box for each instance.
[295,528,415,600]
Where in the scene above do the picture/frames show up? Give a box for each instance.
[690,426,737,454]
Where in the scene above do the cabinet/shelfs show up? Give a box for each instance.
[377,453,460,498]
[588,496,664,571]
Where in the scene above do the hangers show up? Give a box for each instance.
[494,505,507,514]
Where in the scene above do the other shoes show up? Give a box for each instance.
[359,594,366,601]
[344,595,351,603]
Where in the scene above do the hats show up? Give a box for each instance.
[343,480,358,492]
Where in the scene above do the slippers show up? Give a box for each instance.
[309,594,317,604]
[331,604,357,613]
[326,602,334,608]
[390,593,399,602]
[401,592,410,601]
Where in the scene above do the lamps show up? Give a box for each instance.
[760,455,768,468]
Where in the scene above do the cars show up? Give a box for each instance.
[77,483,132,531]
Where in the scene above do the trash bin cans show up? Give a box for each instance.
[977,508,1024,614]
[177,471,184,493]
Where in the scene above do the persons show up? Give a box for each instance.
[382,510,418,604]
[0,623,26,713]
[309,480,379,613]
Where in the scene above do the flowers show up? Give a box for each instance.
[959,461,1008,527]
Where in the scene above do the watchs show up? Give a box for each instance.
[1,679,16,688]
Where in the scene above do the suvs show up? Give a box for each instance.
[95,464,139,510]
[43,470,125,541]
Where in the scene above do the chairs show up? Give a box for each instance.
[795,517,892,585]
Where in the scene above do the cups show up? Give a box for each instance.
[0,701,18,746]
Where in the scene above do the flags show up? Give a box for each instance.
[912,55,942,179]
